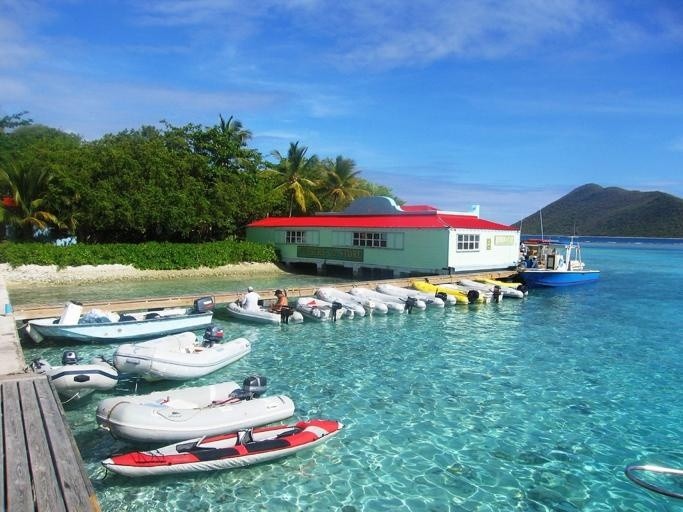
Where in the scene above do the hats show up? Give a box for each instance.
[274,290,283,296]
[248,286,253,292]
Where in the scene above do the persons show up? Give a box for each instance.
[519,241,525,252]
[239,285,262,310]
[268,288,287,313]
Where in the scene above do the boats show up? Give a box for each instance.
[515,209,601,288]
[110,325,255,384]
[288,271,530,324]
[98,417,348,485]
[26,352,120,401]
[22,295,217,344]
[223,296,305,326]
[91,375,302,444]
